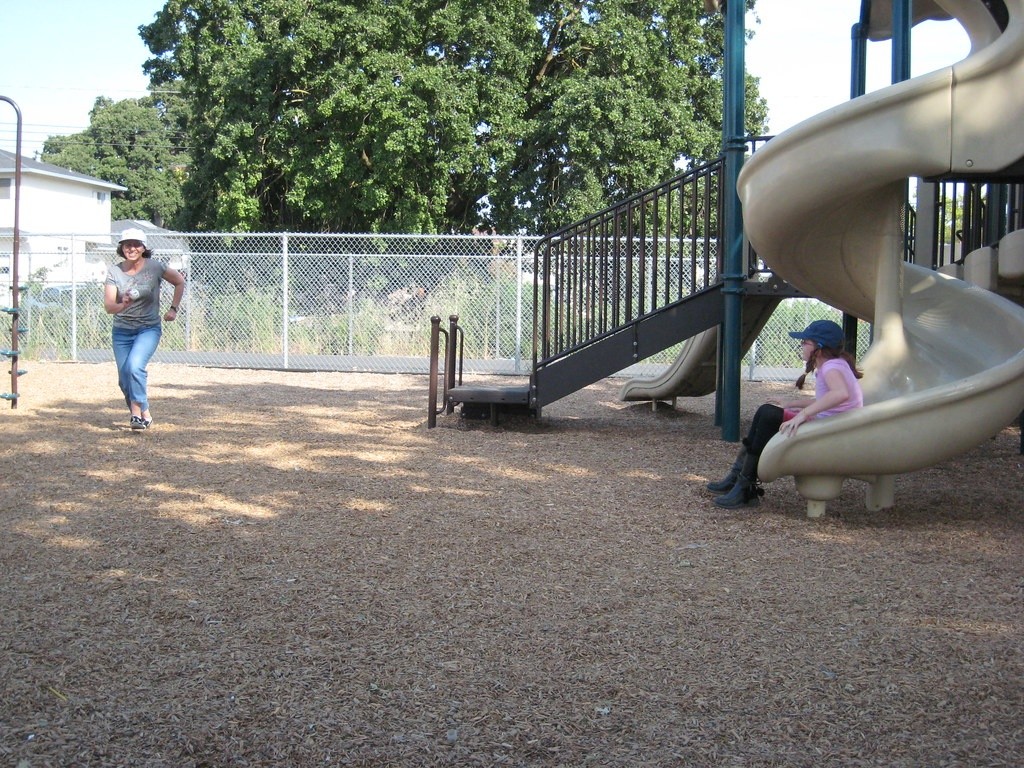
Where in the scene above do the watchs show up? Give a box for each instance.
[170,305,179,313]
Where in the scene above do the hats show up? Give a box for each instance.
[119,228,147,248]
[788,320,845,350]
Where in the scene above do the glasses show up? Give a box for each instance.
[800,340,815,347]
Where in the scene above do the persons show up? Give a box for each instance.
[703,320,863,509]
[104,228,184,432]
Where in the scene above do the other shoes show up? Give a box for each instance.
[130,415,153,430]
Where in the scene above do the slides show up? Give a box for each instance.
[736,0,1024,482]
[620,297,779,406]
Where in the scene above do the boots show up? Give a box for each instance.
[706,438,765,509]
